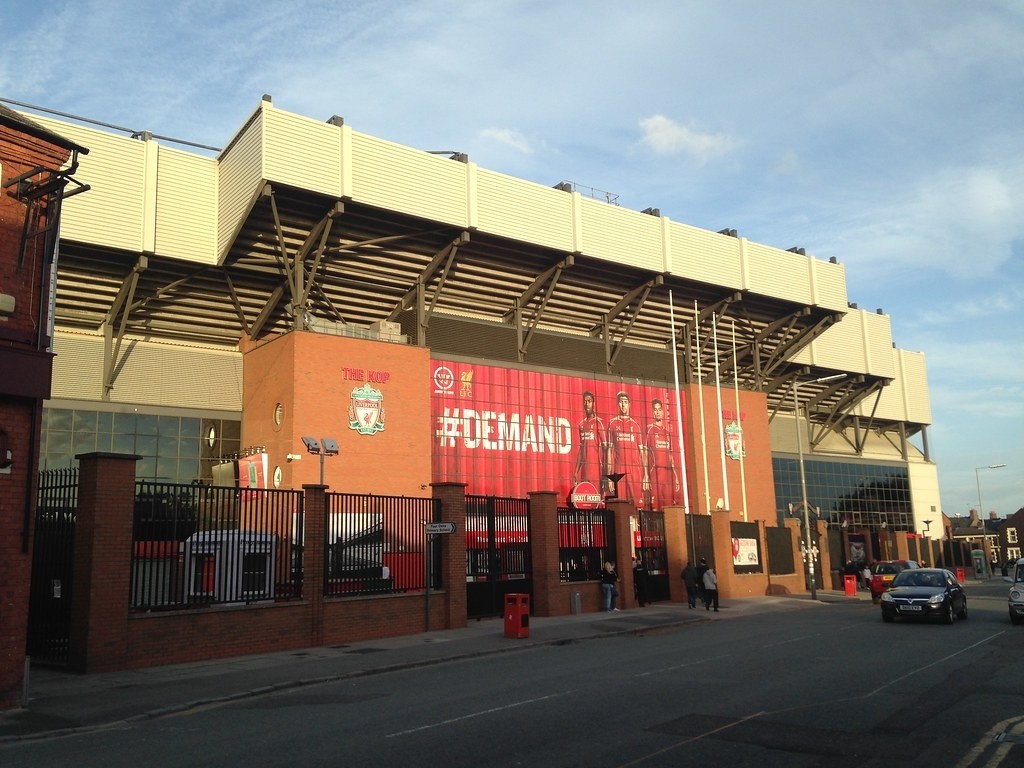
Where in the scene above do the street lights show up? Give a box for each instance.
[975,464,1007,578]
[792,373,849,601]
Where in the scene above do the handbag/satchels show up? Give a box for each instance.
[610,584,618,597]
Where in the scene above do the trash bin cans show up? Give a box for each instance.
[843,575,857,597]
[570,591,582,616]
[955,568,965,583]
[502,593,531,638]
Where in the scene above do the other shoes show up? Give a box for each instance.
[613,608,620,611]
[607,609,614,613]
[714,610,719,612]
[639,604,645,607]
[707,608,709,610]
[688,603,692,609]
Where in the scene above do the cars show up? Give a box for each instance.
[1008,557,1024,625]
[880,567,967,624]
[869,560,923,605]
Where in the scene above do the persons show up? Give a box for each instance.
[857,565,871,590]
[681,558,719,612]
[990,560,999,575]
[572,391,680,512]
[633,559,652,607]
[598,561,621,614]
[1007,554,1019,565]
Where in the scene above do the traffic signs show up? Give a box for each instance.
[424,522,455,534]
